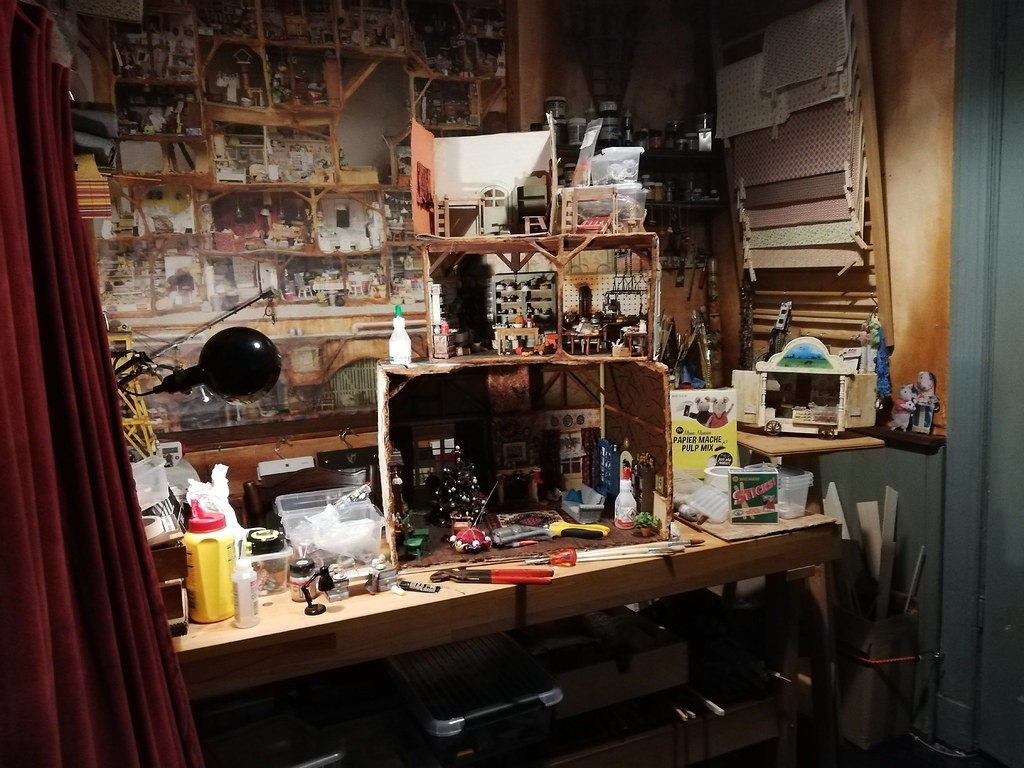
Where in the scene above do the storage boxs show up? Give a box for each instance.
[275,506,385,566]
[276,486,370,517]
[727,468,779,525]
[833,591,919,749]
[128,454,191,637]
[670,389,813,526]
[557,145,649,220]
[190,605,688,768]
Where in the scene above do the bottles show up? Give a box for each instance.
[233,539,260,629]
[615,480,636,529]
[388,318,412,367]
[184,510,238,623]
[624,109,633,146]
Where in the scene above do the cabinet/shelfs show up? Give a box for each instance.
[532,123,730,214]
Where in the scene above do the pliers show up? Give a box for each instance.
[430,567,554,585]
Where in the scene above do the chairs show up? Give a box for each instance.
[449,511,472,535]
[408,509,430,551]
[400,517,423,560]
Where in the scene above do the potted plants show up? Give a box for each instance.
[633,511,654,537]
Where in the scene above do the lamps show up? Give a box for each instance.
[113,325,282,406]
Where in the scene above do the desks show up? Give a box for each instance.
[497,328,538,356]
[571,336,601,355]
[623,334,645,357]
[496,468,536,505]
[155,507,840,768]
